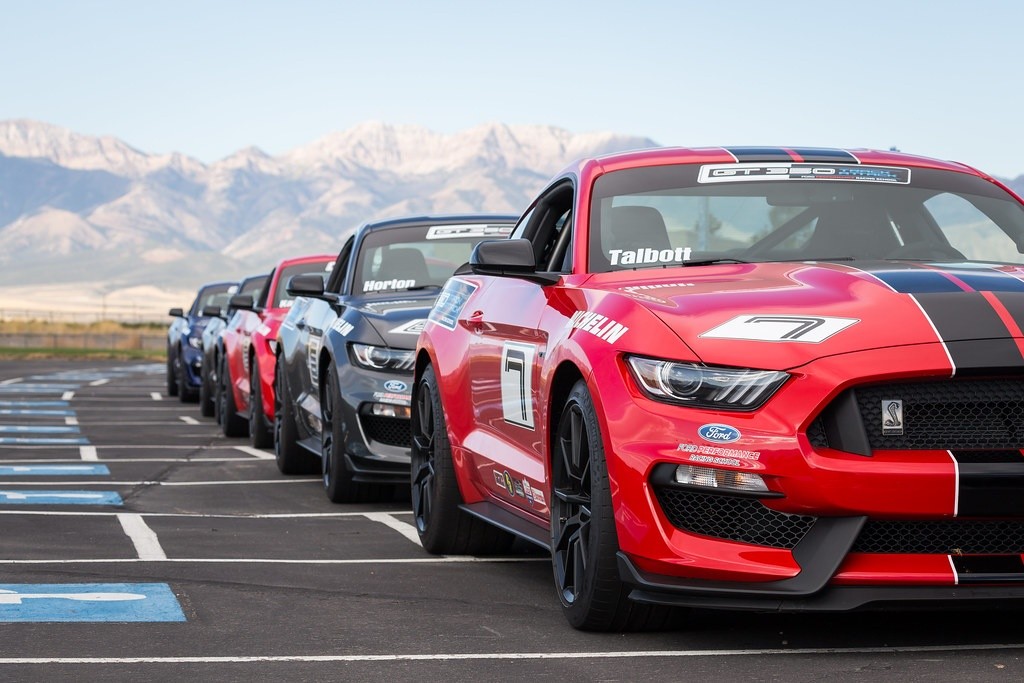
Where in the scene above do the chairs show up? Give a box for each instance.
[563,204,674,271]
[372,246,431,289]
[794,205,906,258]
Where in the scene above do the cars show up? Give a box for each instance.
[166,214,570,506]
[409,146,1024,642]
